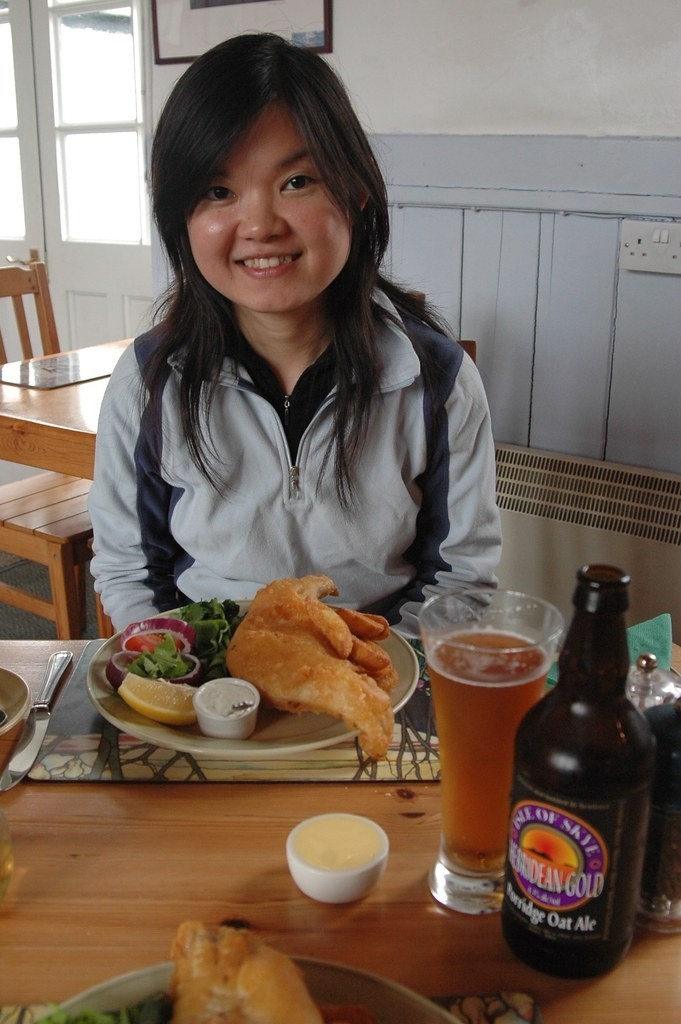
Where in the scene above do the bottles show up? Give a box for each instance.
[501,561,656,976]
[631,696,681,933]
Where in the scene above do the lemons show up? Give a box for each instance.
[117,672,197,725]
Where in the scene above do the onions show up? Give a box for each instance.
[105,649,204,688]
[120,617,197,653]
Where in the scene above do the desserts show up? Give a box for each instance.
[291,815,381,872]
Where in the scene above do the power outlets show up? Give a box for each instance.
[618,221,681,276]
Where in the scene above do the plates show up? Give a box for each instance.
[85,598,419,757]
[0,667,30,736]
[26,955,466,1023]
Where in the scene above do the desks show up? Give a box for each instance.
[0,337,140,482]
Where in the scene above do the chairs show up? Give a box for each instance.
[0,247,97,642]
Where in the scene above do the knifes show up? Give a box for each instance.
[0,649,75,794]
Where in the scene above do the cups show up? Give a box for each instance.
[286,812,389,904]
[417,588,565,913]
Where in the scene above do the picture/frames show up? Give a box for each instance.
[151,0,333,67]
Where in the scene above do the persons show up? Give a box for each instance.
[89,33,504,641]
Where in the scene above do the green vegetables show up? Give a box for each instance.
[18,988,176,1024]
[125,598,248,685]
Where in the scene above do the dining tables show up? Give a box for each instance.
[0,636,681,1024]
